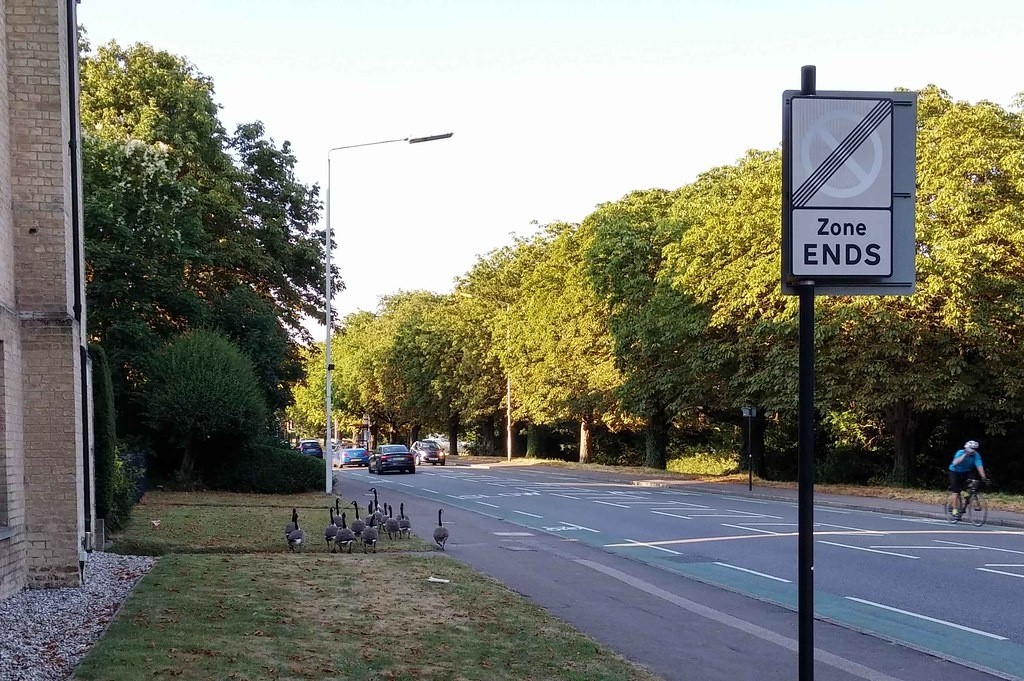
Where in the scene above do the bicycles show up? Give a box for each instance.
[944,479,989,528]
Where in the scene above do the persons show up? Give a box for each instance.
[949,441,988,517]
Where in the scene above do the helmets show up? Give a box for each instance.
[964,441,979,449]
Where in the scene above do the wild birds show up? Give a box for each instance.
[360,514,378,554]
[334,512,357,554]
[433,509,449,551]
[285,508,305,553]
[326,488,411,549]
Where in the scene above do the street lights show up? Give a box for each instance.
[325,131,454,493]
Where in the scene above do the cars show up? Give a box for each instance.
[332,444,368,469]
[410,440,446,466]
[368,445,415,474]
[332,439,355,450]
[295,440,323,458]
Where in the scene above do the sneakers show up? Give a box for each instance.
[952,508,960,516]
[961,491,970,498]
